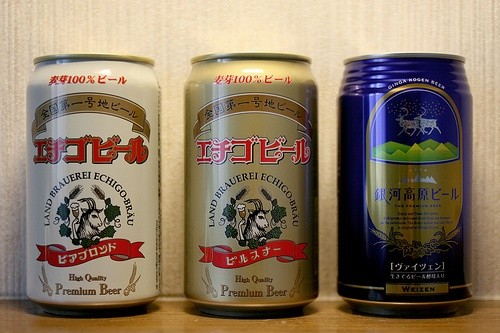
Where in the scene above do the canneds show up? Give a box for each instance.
[336,51,472,311]
[23,54,163,317]
[183,51,319,320]
